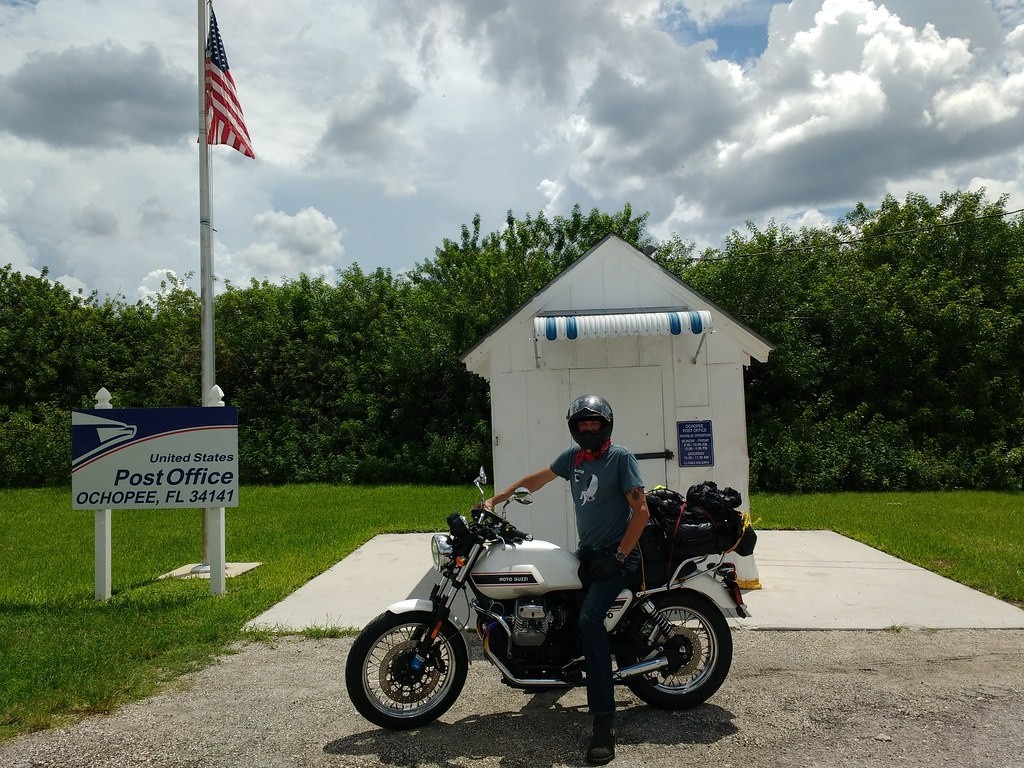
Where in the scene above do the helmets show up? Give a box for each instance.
[567,395,614,450]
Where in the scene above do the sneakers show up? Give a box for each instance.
[588,714,616,764]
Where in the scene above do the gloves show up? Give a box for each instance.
[588,550,624,583]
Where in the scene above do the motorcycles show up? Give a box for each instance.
[344,467,756,728]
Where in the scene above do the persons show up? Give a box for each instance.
[473,393,650,766]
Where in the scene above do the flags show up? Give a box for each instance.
[197,0,256,159]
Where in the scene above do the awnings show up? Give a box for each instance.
[533,311,714,369]
